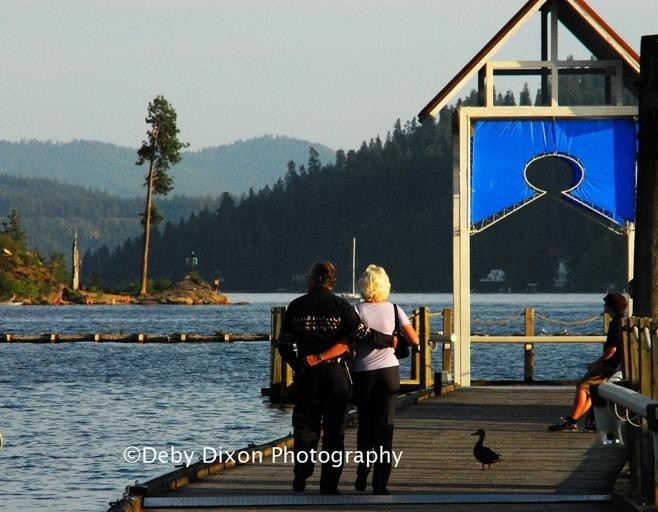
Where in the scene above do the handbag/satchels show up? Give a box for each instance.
[393,328,409,360]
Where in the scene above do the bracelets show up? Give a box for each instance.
[318,354,323,360]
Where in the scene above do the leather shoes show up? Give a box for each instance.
[372,487,390,495]
[292,466,306,493]
[354,467,369,491]
[320,489,343,495]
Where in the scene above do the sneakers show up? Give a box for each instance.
[547,421,578,433]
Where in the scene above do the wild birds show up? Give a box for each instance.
[470,427,503,470]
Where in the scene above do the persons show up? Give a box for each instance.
[213,278,219,294]
[278,260,398,495]
[628,279,634,298]
[305,264,419,495]
[550,292,627,432]
